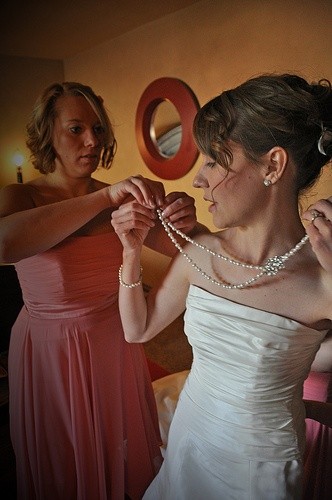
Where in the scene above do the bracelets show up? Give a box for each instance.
[119,265,144,288]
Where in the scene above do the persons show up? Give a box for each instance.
[0,83,212,500]
[111,73,332,500]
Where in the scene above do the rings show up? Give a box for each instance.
[185,206,194,218]
[312,213,324,223]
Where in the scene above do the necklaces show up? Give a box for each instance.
[156,209,310,289]
